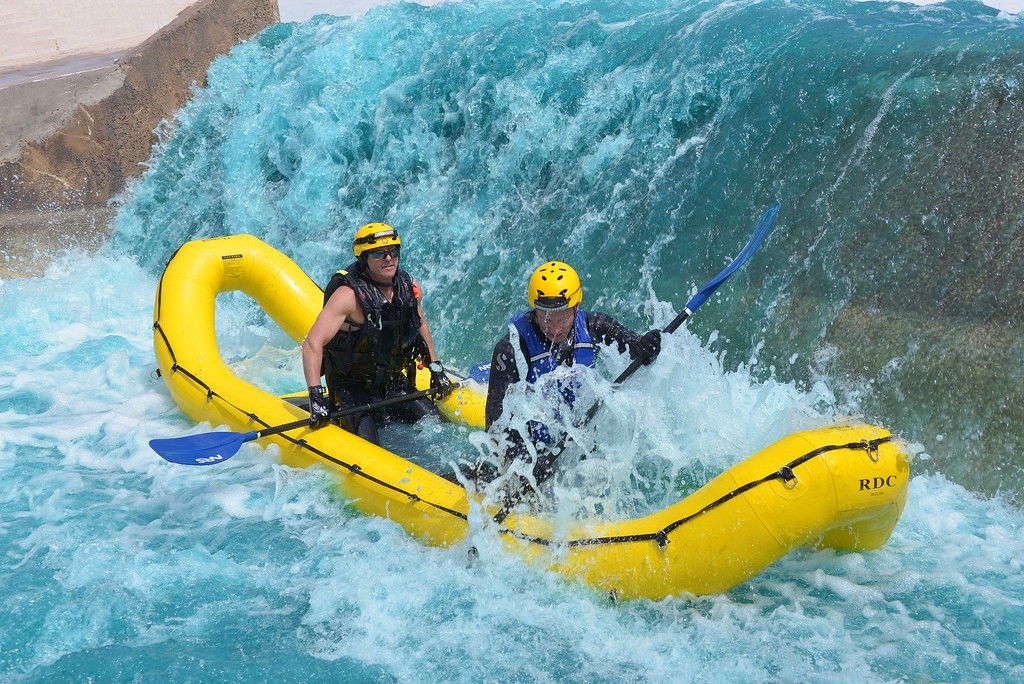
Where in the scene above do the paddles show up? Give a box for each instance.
[148,360,492,467]
[468,202,783,558]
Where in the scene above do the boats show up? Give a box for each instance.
[151,231,912,602]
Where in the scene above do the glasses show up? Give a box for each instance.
[363,247,400,261]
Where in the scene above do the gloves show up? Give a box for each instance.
[631,328,661,367]
[428,360,454,402]
[308,385,332,430]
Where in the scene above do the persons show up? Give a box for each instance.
[302,222,455,446]
[482,261,660,479]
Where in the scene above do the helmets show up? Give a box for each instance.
[353,222,401,257]
[528,262,582,310]
